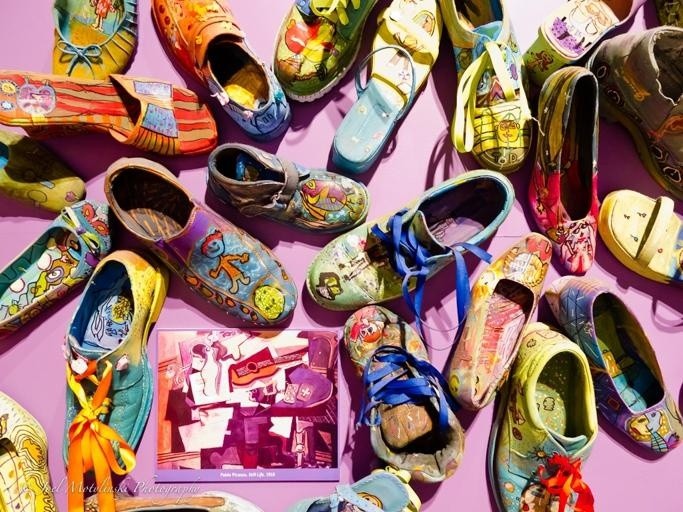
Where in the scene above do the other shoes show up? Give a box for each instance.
[272,1,683,512]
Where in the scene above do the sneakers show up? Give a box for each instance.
[85,491,265,512]
[57,249,171,480]
[205,142,370,232]
[0,199,116,340]
[152,1,292,142]
[104,157,298,326]
[1,391,57,512]
[52,2,139,80]
[0,130,86,213]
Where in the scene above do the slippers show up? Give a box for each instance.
[1,69,219,156]
